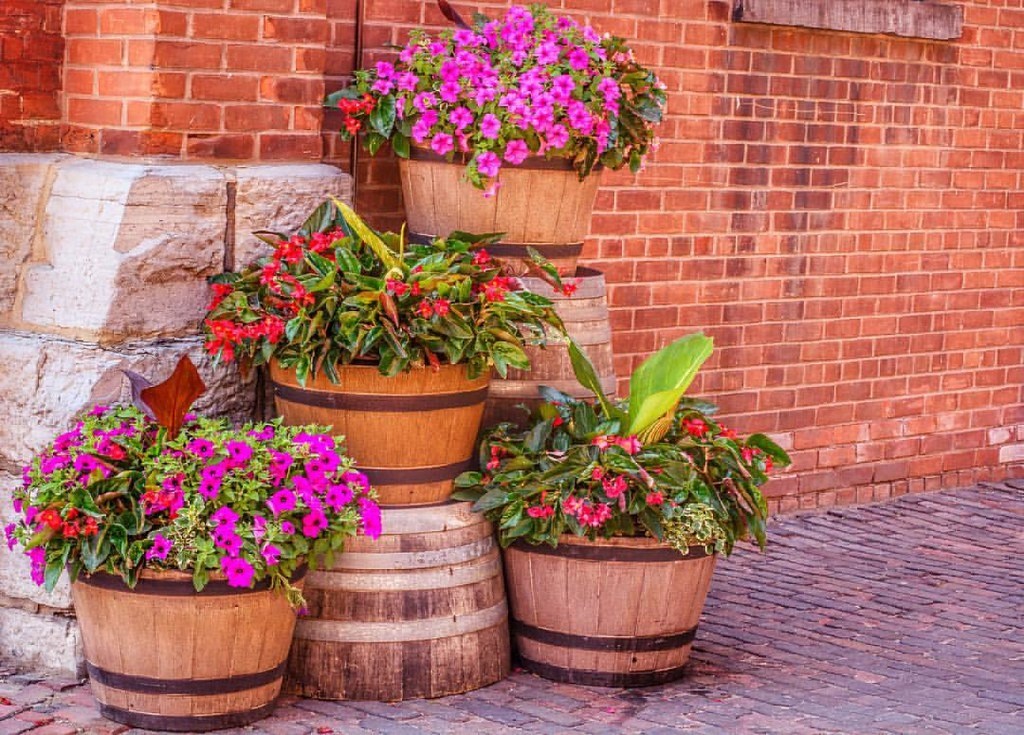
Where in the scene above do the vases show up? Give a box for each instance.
[399,137,604,280]
[502,531,718,687]
[66,556,307,730]
[270,354,492,509]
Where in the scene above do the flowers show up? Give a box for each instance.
[447,328,793,556]
[198,191,582,377]
[5,353,382,617]
[315,3,672,198]
